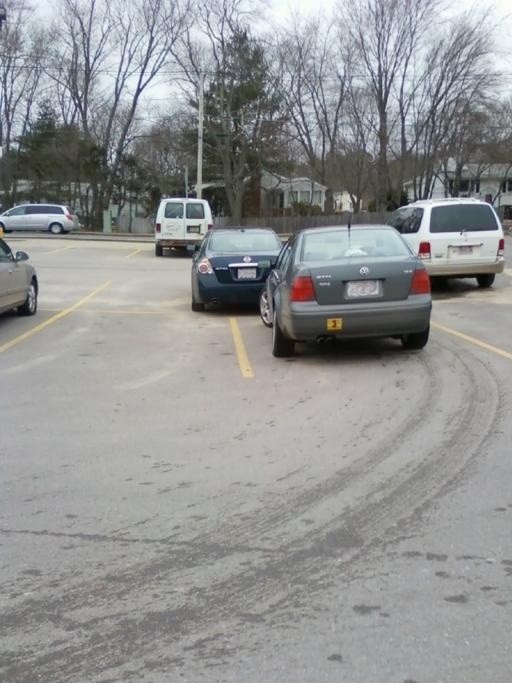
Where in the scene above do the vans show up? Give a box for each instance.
[382,197,506,288]
[152,196,215,257]
[0,203,80,234]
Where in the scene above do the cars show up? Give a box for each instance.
[0,235,40,316]
[186,224,285,312]
[257,209,434,361]
